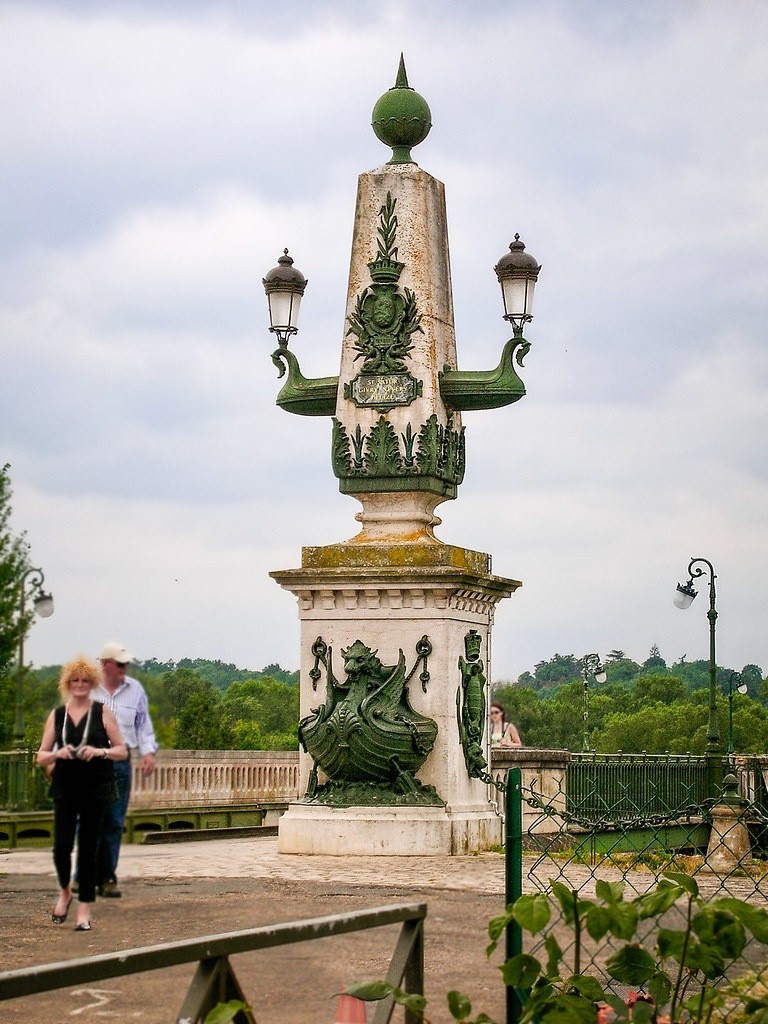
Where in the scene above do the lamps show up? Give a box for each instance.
[260,247,310,350]
[492,232,542,338]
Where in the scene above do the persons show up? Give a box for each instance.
[68,642,158,898]
[488,704,521,747]
[36,658,128,932]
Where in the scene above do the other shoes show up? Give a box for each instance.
[52,894,73,922]
[73,919,91,930]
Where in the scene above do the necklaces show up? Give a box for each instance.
[62,693,92,750]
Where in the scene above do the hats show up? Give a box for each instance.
[100,641,131,664]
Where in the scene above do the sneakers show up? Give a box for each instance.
[71,880,81,893]
[97,878,122,898]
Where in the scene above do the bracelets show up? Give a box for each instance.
[100,747,109,761]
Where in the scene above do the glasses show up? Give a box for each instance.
[107,661,128,668]
[491,711,499,715]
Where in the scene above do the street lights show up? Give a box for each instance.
[9,569,54,809]
[582,652,608,753]
[726,671,747,755]
[673,558,720,803]
